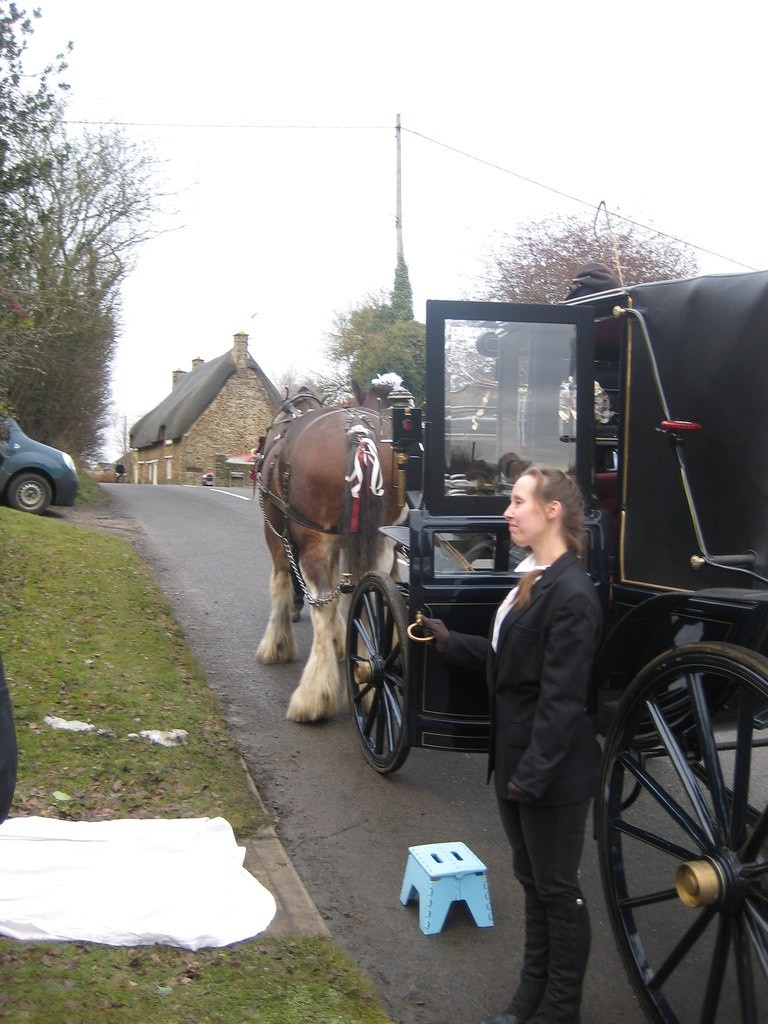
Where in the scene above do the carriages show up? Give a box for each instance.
[255,271,766,1023]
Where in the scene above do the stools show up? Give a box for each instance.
[399,841,494,935]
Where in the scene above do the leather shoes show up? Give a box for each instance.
[479,1012,528,1024]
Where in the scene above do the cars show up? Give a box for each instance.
[0,415,80,516]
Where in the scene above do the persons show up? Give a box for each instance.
[115,461,127,484]
[420,464,607,1024]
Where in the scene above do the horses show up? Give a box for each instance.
[252,385,413,727]
